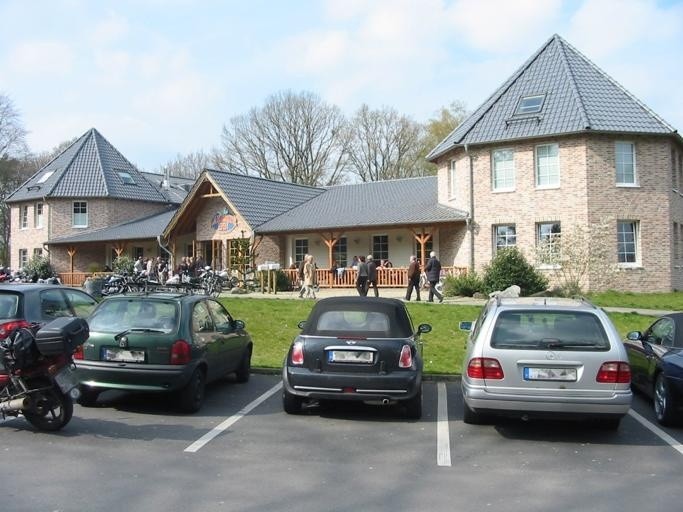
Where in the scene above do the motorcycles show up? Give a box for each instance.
[0,263,63,283]
[0,316,90,432]
[101,265,240,295]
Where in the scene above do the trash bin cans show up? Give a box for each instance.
[86,277,102,298]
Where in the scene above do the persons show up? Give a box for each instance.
[134,256,204,283]
[365,255,379,297]
[297,254,308,298]
[351,255,358,266]
[403,255,421,302]
[304,255,316,299]
[355,255,368,296]
[376,260,385,268]
[289,261,299,269]
[423,251,444,303]
[103,265,113,272]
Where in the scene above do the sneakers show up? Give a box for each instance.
[426,297,444,303]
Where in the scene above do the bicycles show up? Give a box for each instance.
[418,264,450,295]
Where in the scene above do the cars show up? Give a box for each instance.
[0,282,254,416]
[282,296,432,419]
[459,295,683,431]
